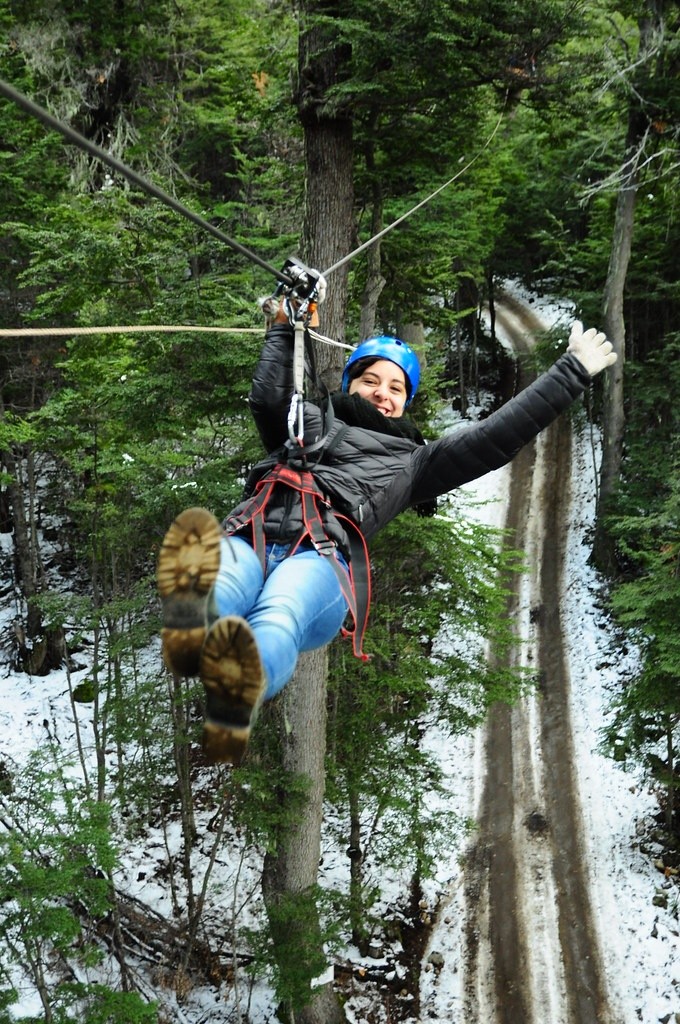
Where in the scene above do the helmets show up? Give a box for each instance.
[341,335,421,408]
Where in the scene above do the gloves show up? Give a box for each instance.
[566,319,618,377]
[311,268,327,307]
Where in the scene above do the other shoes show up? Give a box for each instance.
[197,615,266,770]
[154,506,223,679]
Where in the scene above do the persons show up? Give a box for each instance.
[158,269,618,765]
